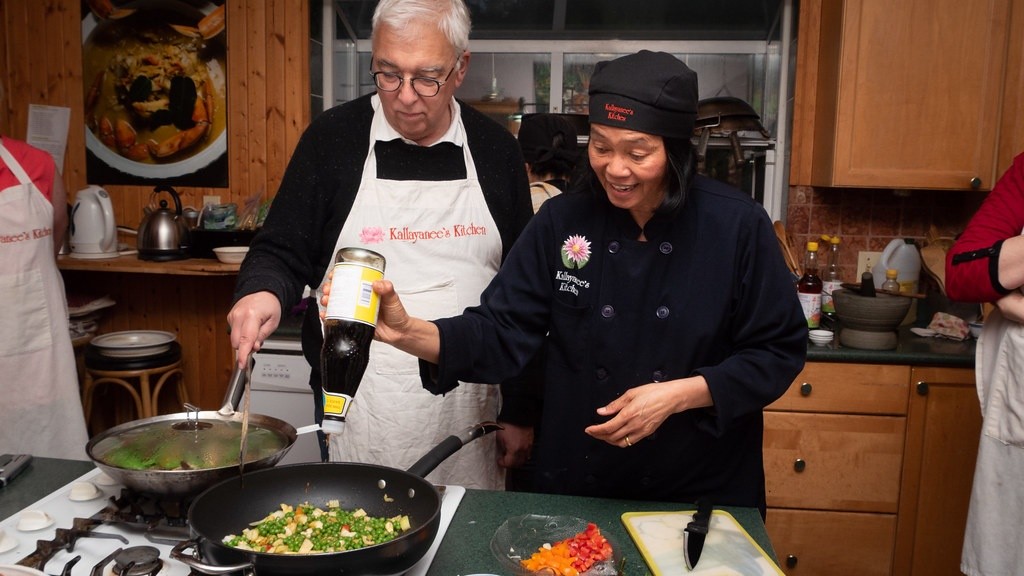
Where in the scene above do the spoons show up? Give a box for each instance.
[774,221,800,276]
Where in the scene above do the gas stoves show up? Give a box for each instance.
[0,467,466,576]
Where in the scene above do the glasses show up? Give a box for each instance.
[368,47,466,98]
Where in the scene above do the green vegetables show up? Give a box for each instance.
[105,429,274,470]
[126,72,198,129]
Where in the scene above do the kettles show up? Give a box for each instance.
[137,184,196,261]
[69,185,119,259]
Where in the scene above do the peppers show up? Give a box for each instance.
[520,523,613,576]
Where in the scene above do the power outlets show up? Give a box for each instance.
[855,250,883,283]
[202,195,221,208]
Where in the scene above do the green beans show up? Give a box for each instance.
[225,505,402,554]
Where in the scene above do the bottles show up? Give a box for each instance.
[882,270,899,293]
[872,238,922,325]
[822,235,845,317]
[183,211,199,231]
[322,248,386,435]
[508,113,522,139]
[798,242,823,344]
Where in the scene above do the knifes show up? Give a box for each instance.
[684,498,715,571]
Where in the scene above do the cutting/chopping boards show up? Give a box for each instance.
[621,510,787,576]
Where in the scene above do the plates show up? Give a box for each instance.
[82,3,227,178]
[91,330,176,357]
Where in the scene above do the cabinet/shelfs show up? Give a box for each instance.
[762,362,911,576]
[810,0,1024,191]
[892,366,984,576]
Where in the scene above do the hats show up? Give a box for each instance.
[518,113,580,165]
[587,48,698,140]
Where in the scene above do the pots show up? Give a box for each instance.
[85,357,298,502]
[170,421,504,576]
[694,97,770,165]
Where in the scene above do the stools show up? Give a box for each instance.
[80,342,191,430]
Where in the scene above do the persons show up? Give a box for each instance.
[943,150,1024,576]
[0,135,93,462]
[226,0,538,491]
[317,49,808,516]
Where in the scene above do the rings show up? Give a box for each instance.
[625,437,633,449]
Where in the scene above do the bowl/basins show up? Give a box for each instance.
[809,330,833,347]
[490,514,621,576]
[213,247,250,264]
[0,564,49,576]
[969,321,984,340]
[184,229,262,259]
